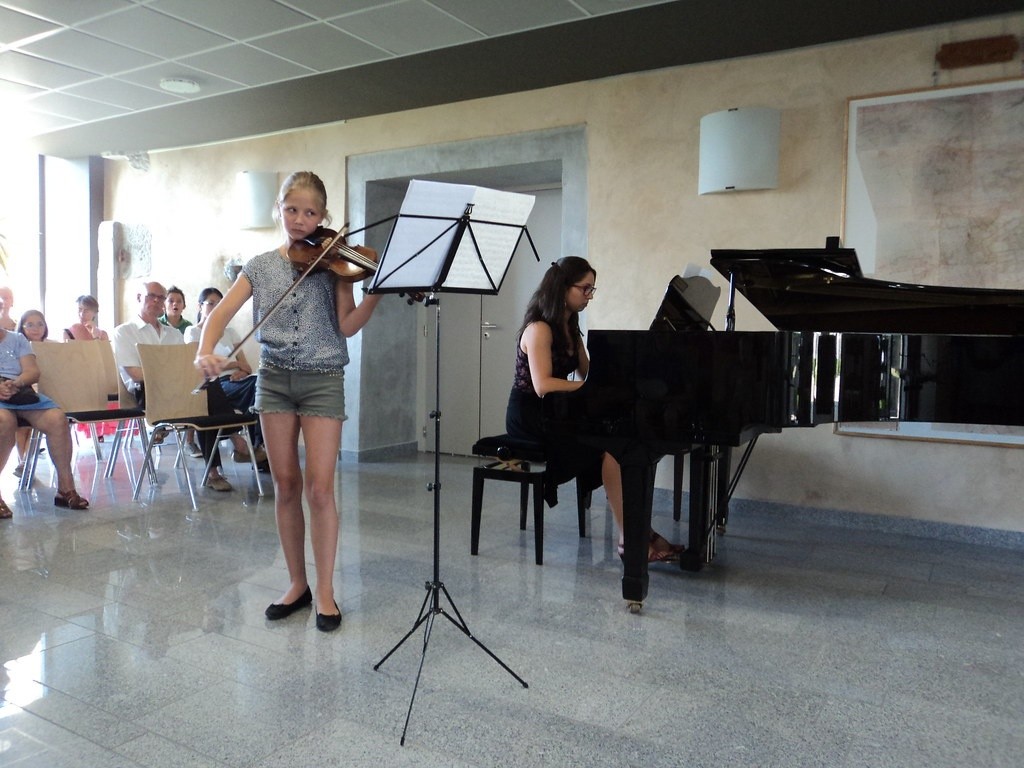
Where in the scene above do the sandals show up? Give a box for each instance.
[651,533,685,554]
[618,540,669,568]
[54,489,90,509]
[0,497,12,518]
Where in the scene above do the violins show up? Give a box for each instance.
[288,226,425,307]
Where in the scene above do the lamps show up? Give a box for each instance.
[696,105,784,196]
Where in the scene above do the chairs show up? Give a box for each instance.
[103,347,160,493]
[130,340,266,512]
[18,340,149,507]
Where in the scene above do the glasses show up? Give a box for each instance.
[141,294,167,302]
[22,323,45,330]
[572,284,596,296]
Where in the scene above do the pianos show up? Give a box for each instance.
[581,234,1024,613]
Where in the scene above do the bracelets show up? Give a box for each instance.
[16,377,25,386]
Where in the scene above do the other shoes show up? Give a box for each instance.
[252,459,271,473]
[152,434,164,445]
[12,465,30,478]
[205,477,231,490]
[316,599,342,632]
[234,447,259,463]
[266,586,314,619]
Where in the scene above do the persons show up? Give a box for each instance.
[1,303,92,520]
[146,286,202,455]
[63,295,140,443]
[111,279,269,491]
[194,171,385,630]
[9,307,48,481]
[0,287,19,335]
[184,288,268,469]
[504,257,686,566]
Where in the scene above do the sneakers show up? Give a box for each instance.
[182,442,203,458]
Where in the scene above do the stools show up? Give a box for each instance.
[470,433,588,566]
